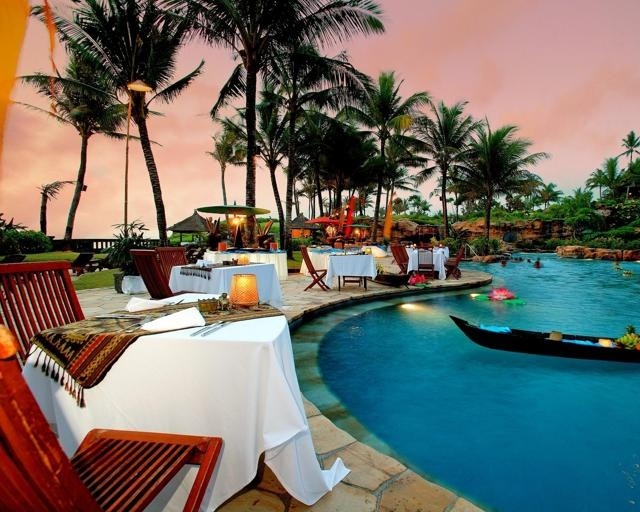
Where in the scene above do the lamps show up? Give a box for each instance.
[228,273,261,309]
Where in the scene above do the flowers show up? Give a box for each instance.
[488,286,516,300]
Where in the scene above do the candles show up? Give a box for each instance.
[111,220,149,292]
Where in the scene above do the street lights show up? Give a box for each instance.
[123,76,158,238]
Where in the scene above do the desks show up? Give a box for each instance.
[18,292,349,511]
[169,247,288,306]
[128,234,208,300]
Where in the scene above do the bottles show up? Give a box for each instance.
[219,293,229,311]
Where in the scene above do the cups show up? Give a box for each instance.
[270,243,277,251]
[236,252,252,265]
[218,242,226,251]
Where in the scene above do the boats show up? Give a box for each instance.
[447,313,640,365]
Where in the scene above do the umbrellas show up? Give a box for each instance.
[196,201,271,218]
[304,217,346,224]
[349,224,372,228]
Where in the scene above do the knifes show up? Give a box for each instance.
[191,321,230,337]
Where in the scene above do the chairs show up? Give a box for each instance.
[2,323,224,511]
[300,244,464,292]
[69,253,106,277]
[0,260,85,370]
[0,254,27,263]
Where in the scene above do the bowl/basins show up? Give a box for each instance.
[198,298,218,312]
